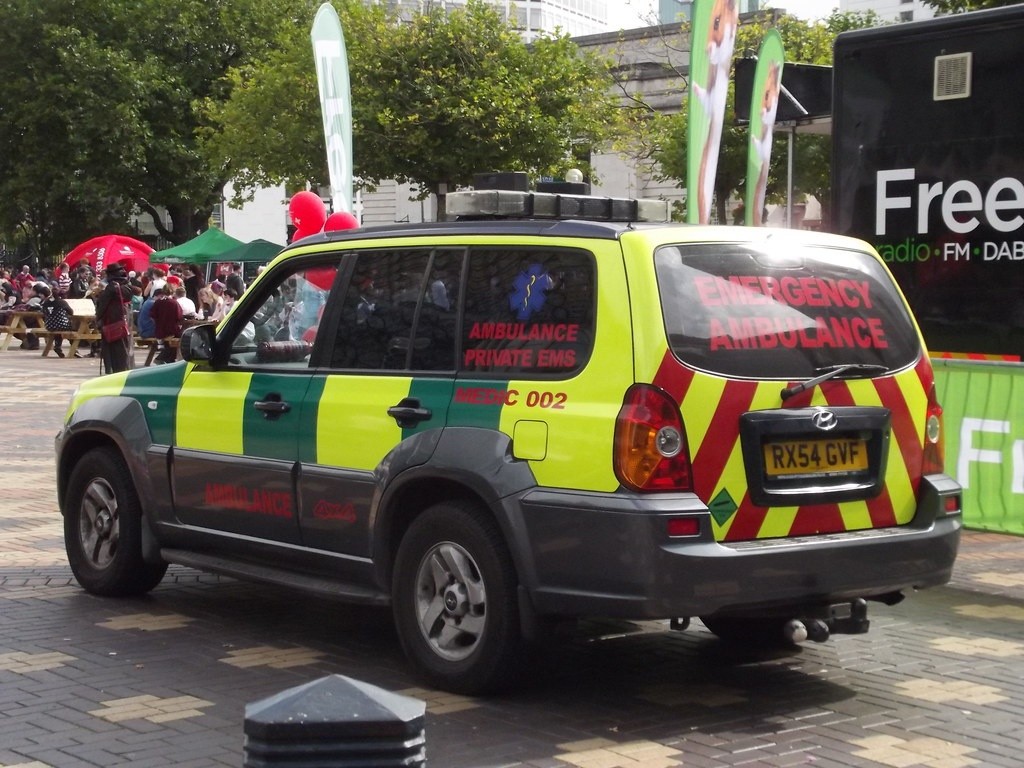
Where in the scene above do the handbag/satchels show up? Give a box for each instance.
[102,281,130,343]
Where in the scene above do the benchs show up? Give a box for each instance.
[0,325,182,358]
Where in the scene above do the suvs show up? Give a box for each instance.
[51,187,964,698]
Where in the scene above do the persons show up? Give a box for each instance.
[0,259,269,365]
[95,263,136,374]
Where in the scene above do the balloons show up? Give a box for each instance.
[289,191,372,340]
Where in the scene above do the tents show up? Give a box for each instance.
[54,226,286,285]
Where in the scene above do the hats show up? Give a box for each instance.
[102,263,125,271]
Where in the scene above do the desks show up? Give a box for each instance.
[43,314,99,358]
[2,311,55,349]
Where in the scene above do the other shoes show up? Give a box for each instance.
[75,351,83,357]
[54,347,64,358]
[84,353,95,357]
[153,358,165,364]
[20,343,39,349]
